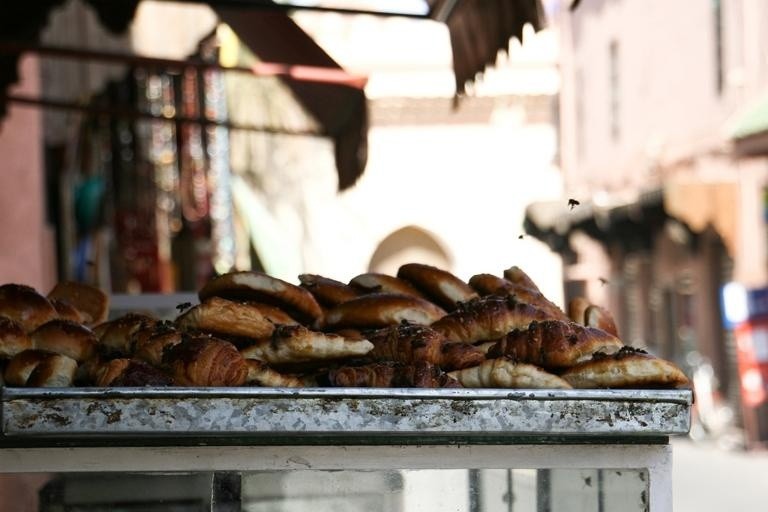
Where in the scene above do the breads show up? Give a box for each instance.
[0,263,689,389]
